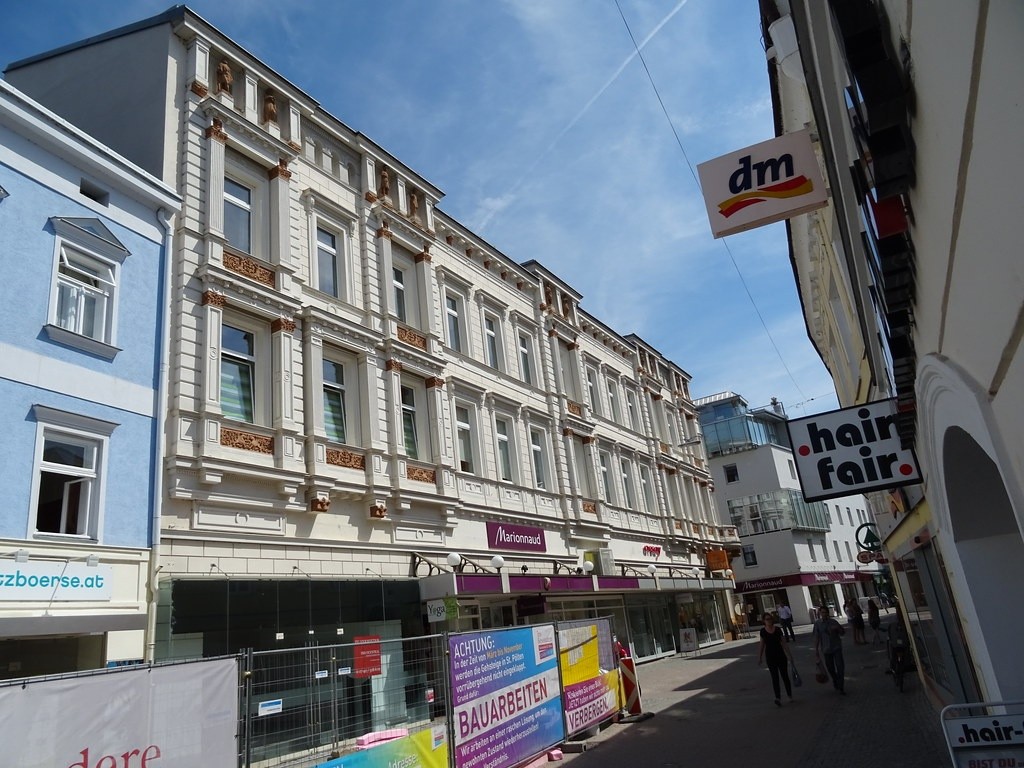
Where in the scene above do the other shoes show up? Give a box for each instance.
[787,695,794,703]
[840,690,848,698]
[775,698,781,706]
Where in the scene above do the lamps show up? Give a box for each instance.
[68,554,99,567]
[491,555,504,573]
[692,567,700,578]
[447,552,461,571]
[725,569,733,579]
[647,565,657,577]
[0,549,29,563]
[583,561,594,575]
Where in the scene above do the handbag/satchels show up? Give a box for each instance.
[816,658,828,684]
[790,662,802,687]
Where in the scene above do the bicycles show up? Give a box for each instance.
[877,623,909,693]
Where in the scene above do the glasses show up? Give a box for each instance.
[765,618,773,621]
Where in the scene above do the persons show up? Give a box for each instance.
[409,189,418,218]
[546,284,552,306]
[676,375,680,389]
[811,606,845,694]
[216,58,233,98]
[757,612,795,706]
[777,600,796,642]
[640,350,645,367]
[381,167,390,196]
[264,88,278,122]
[843,590,899,646]
[563,295,569,318]
